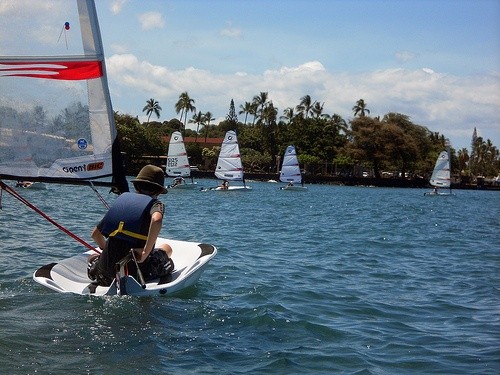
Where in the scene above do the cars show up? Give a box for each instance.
[382,172,423,180]
[363,172,369,178]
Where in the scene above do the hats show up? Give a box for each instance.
[129,165,168,194]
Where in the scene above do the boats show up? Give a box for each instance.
[15,180,46,190]
[267,179,277,183]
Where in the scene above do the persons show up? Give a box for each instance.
[222,180,228,187]
[288,181,294,186]
[174,180,181,186]
[91,164,173,282]
[433,187,437,192]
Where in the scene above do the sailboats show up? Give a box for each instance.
[165,131,198,188]
[208,130,253,191]
[424,150,456,196]
[279,145,308,191]
[0,0,217,299]
